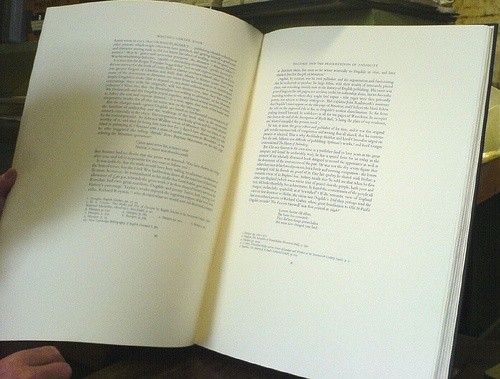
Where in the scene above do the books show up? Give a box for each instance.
[0,2,495,379]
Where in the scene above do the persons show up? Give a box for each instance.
[0,344,69,379]
